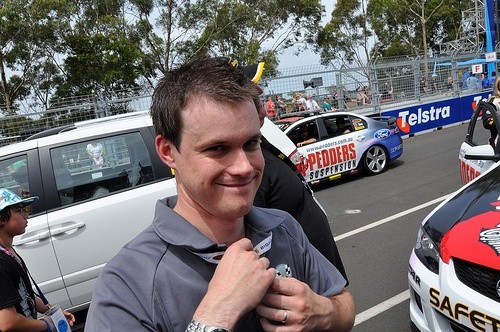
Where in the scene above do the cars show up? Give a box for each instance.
[273,110,403,186]
[405,101,500,332]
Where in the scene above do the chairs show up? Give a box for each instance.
[54,167,74,206]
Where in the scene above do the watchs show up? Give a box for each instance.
[185,320,230,332]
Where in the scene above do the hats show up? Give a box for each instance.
[0,187,40,210]
[228,56,265,83]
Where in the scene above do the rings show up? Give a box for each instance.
[280,310,289,322]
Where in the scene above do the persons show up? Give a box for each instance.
[87,140,105,164]
[63,145,82,170]
[445,70,497,89]
[356,83,394,107]
[231,59,349,287]
[0,188,76,332]
[420,74,439,93]
[481,76,500,145]
[265,91,348,117]
[84,55,358,332]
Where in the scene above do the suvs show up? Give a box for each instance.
[0,111,309,318]
